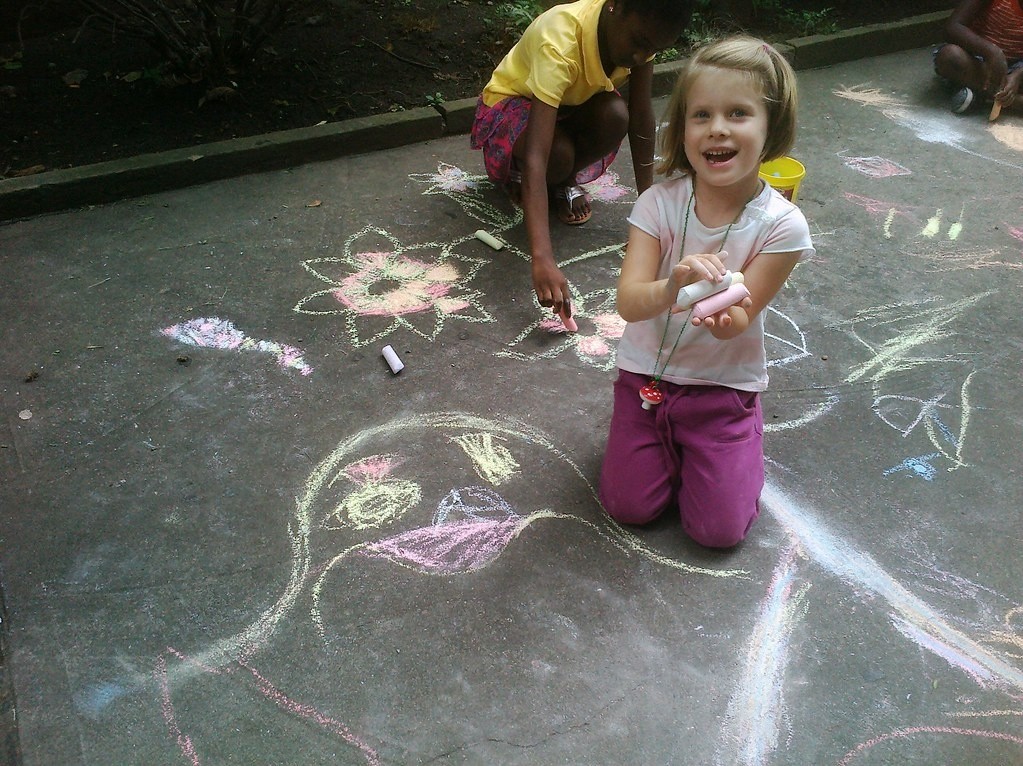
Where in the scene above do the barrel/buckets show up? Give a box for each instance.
[758,154,807,203]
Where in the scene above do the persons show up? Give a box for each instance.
[469,0,694,318]
[600,34,817,548]
[933,0,1023,113]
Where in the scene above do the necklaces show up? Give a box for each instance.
[639,183,760,408]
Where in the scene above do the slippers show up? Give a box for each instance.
[504,161,524,206]
[553,183,592,225]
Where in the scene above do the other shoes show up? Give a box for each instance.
[945,78,983,112]
